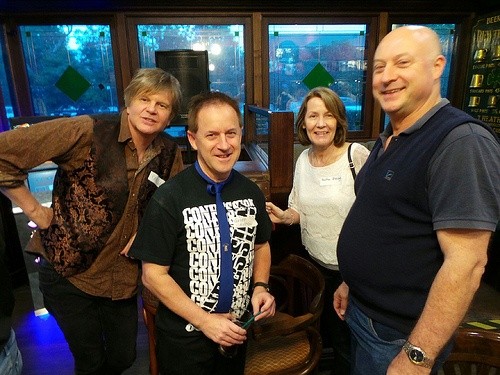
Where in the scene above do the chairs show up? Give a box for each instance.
[142,254,325,375]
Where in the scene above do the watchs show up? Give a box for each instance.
[400,341,436,370]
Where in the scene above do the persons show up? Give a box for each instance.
[331,24,500,375]
[132,89,276,375]
[0,68,201,374]
[0,192,34,374]
[262,85,384,375]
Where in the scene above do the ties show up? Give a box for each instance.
[195,161,235,313]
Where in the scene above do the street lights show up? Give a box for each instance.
[335,81,341,95]
[355,79,360,106]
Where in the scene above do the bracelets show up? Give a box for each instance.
[285,209,294,226]
[251,281,273,293]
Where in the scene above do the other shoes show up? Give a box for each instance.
[252,311,314,342]
[331,365,347,375]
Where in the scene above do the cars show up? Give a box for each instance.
[50,103,86,117]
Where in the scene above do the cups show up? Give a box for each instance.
[220,309,254,354]
